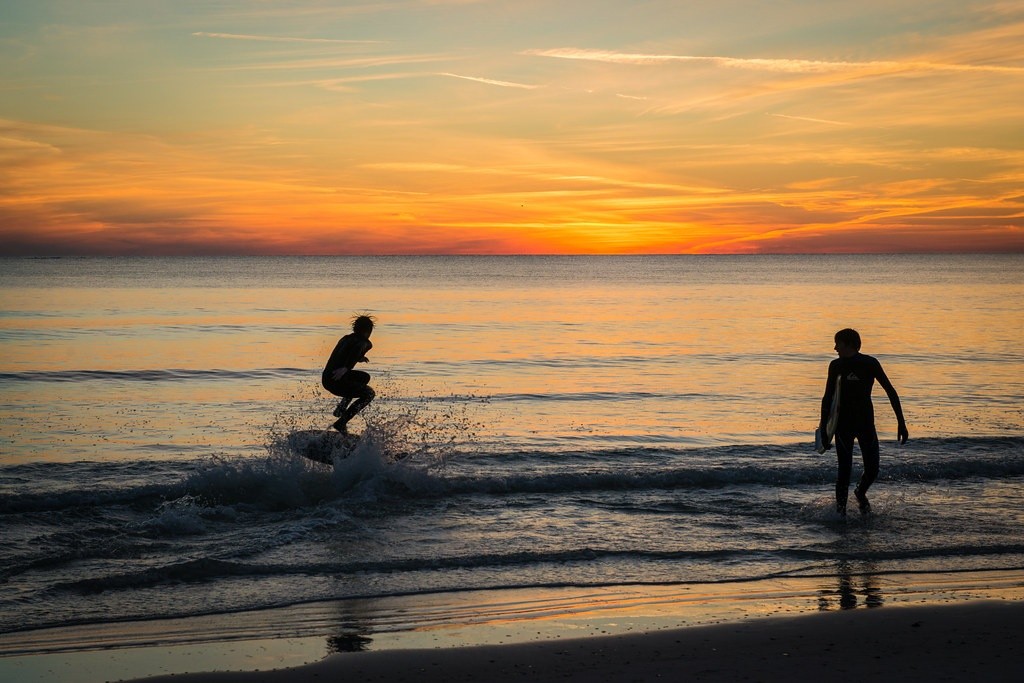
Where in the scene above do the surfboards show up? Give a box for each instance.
[284,429,362,466]
[815,373,846,455]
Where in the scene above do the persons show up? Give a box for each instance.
[820,328,909,515]
[321,314,376,437]
